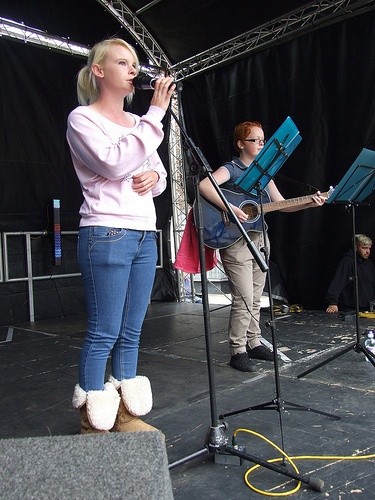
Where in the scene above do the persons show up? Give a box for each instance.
[199,122,328,372]
[326,234,375,313]
[66,38,176,435]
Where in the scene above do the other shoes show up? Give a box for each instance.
[229,352,256,372]
[246,345,281,362]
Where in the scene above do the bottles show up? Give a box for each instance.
[365,331,375,362]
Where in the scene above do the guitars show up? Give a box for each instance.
[193,186,337,249]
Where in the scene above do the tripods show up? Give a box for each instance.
[166,83,326,490]
[295,145,375,380]
[219,115,341,465]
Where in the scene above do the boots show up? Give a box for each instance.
[107,374,159,433]
[71,381,121,435]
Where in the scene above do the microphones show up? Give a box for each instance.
[133,75,183,91]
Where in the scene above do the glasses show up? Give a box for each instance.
[244,138,265,143]
